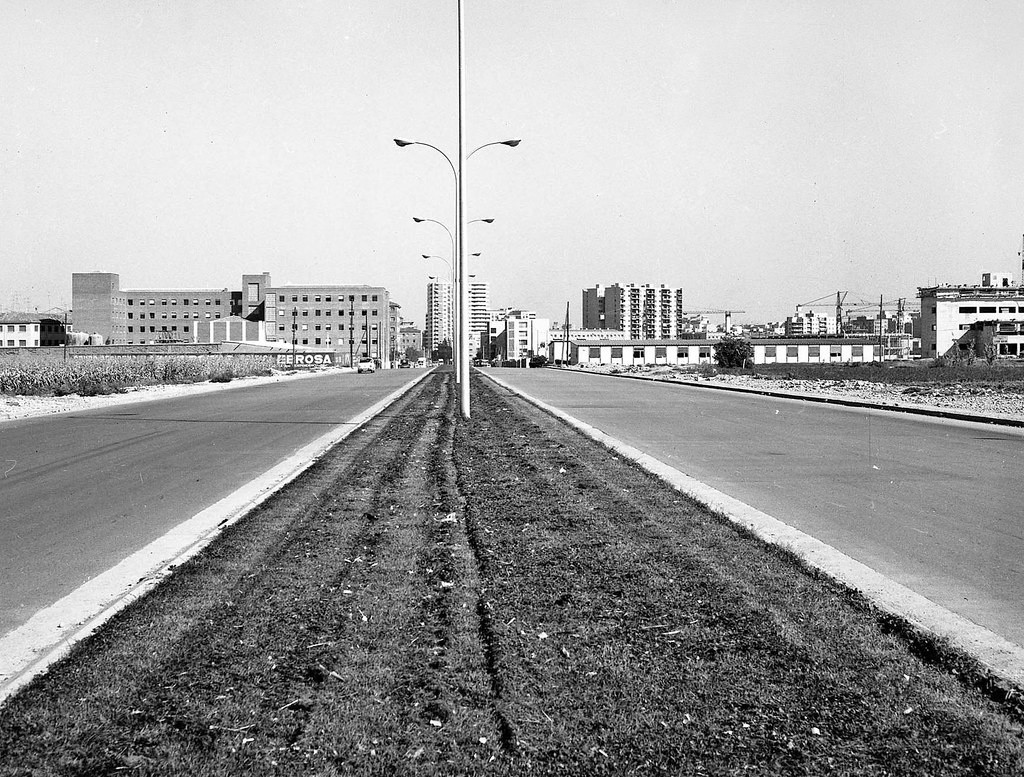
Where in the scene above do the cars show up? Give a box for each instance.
[357,357,375,373]
[438,359,444,366]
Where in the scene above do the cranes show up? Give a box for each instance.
[795,291,880,337]
[845,297,923,333]
[682,306,746,315]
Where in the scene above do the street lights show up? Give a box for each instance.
[421,252,483,366]
[413,217,495,368]
[393,138,521,385]
